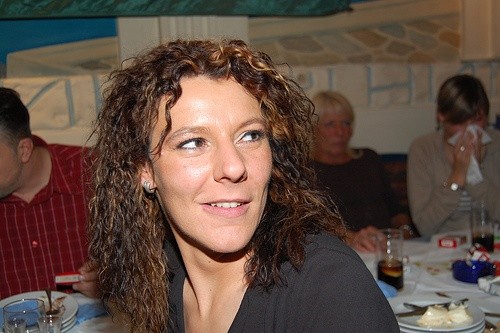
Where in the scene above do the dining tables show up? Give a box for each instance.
[64,225,500,333]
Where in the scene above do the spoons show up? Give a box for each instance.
[45,289,60,315]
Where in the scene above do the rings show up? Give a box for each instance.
[459,145,466,152]
[364,233,369,239]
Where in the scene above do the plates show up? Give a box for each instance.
[393,300,486,333]
[0,290,79,333]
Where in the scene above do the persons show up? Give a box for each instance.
[407,74,500,237]
[301,89,409,252]
[84,39,401,333]
[0,84,102,299]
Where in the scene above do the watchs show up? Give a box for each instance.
[447,181,465,192]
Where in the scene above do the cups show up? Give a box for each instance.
[3,298,45,333]
[471,198,494,253]
[375,229,403,291]
[37,315,62,333]
